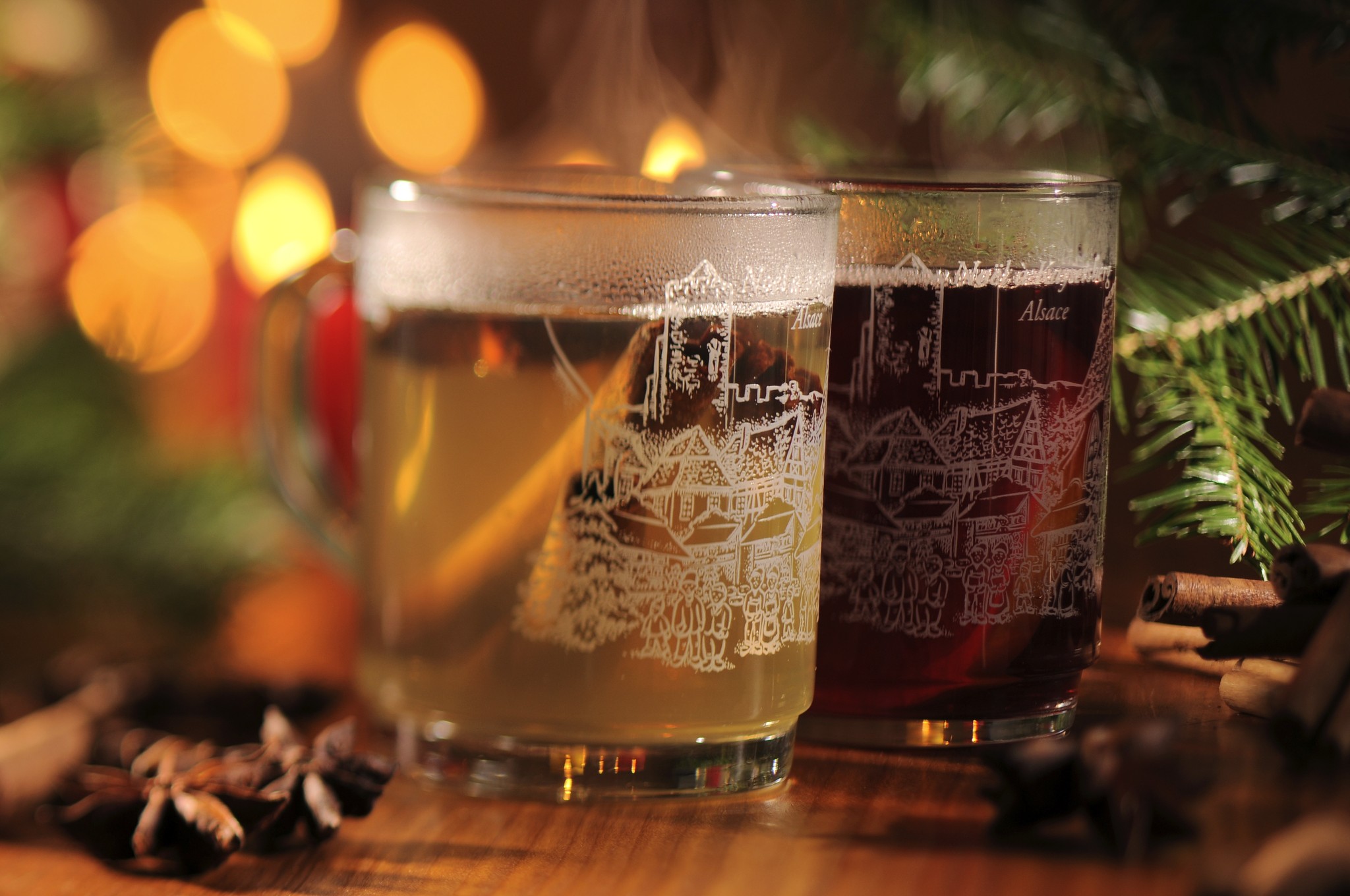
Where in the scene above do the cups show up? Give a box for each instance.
[249,158,841,805]
[768,161,1120,749]
